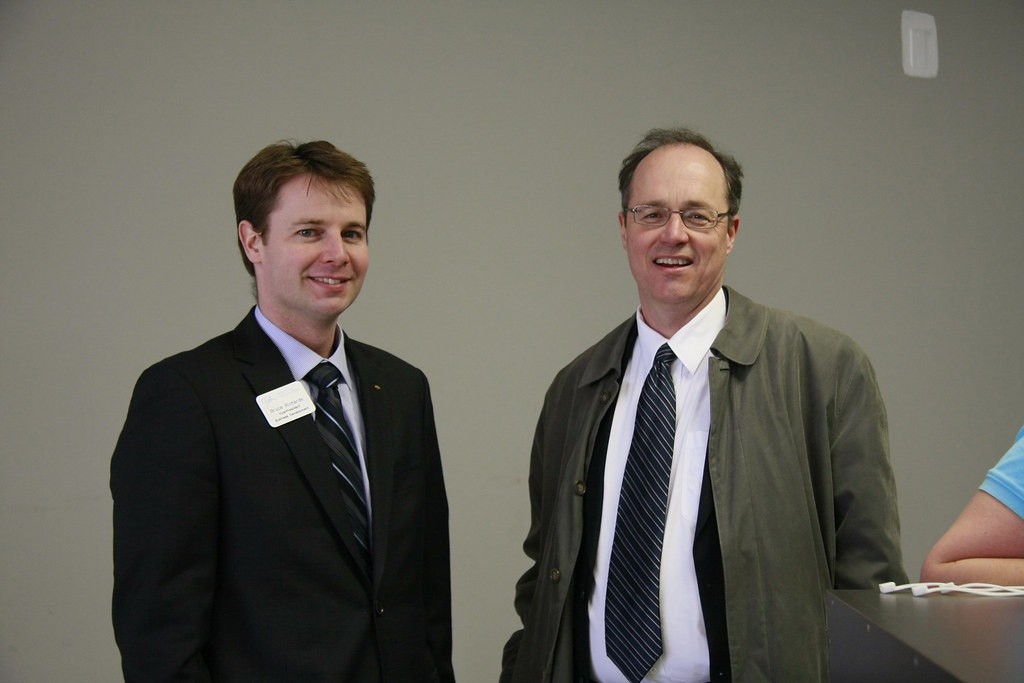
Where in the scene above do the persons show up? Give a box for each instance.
[498,127,911,683]
[919,423,1024,589]
[110,141,457,683]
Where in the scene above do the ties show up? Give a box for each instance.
[303,360,370,561]
[605,342,679,683]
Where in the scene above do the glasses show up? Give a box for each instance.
[626,204,731,229]
